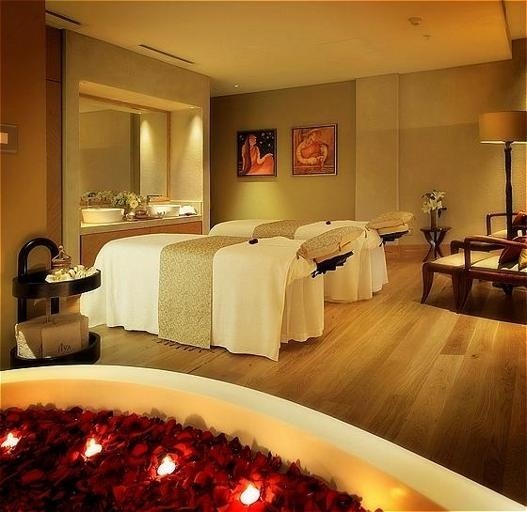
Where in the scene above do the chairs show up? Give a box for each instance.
[450,211,526,256]
[421,225,527,314]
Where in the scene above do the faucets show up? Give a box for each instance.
[87,197,96,207]
[147,194,163,203]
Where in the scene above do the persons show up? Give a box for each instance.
[241,134,273,175]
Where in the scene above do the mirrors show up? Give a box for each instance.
[79,92,172,206]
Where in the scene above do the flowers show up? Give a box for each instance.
[111,191,142,208]
[418,189,447,214]
[81,191,111,206]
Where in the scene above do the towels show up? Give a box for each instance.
[14,313,90,359]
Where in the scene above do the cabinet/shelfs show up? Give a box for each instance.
[9,238,101,370]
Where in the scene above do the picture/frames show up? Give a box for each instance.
[291,123,338,177]
[236,128,277,177]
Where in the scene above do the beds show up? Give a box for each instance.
[97,234,355,355]
[207,221,408,306]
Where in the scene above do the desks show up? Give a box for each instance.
[420,225,452,262]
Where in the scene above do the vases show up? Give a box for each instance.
[430,209,438,229]
[123,209,135,221]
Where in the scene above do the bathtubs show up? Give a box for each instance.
[0,363,524,510]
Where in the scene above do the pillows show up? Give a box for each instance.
[365,211,414,230]
[295,225,364,262]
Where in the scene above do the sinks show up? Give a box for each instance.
[82,208,124,222]
[146,204,181,216]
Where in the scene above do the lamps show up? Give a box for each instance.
[478,110,527,295]
